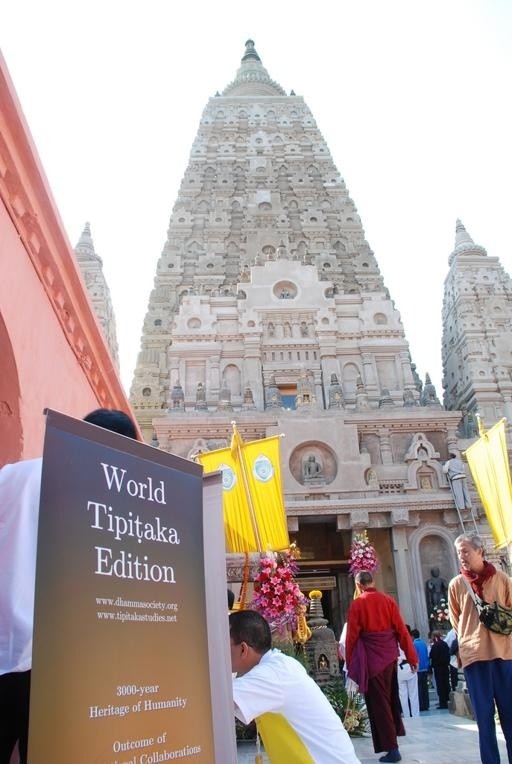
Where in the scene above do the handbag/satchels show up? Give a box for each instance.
[476,598,512,638]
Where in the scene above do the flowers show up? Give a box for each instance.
[428,597,451,629]
[250,557,310,635]
[346,532,381,579]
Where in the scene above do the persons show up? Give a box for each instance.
[228,610,363,763]
[304,455,324,478]
[394,624,462,718]
[338,620,357,697]
[425,566,452,631]
[446,533,512,763]
[344,570,420,763]
[442,451,472,513]
[1,406,139,763]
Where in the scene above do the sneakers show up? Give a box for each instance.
[379,752,402,763]
